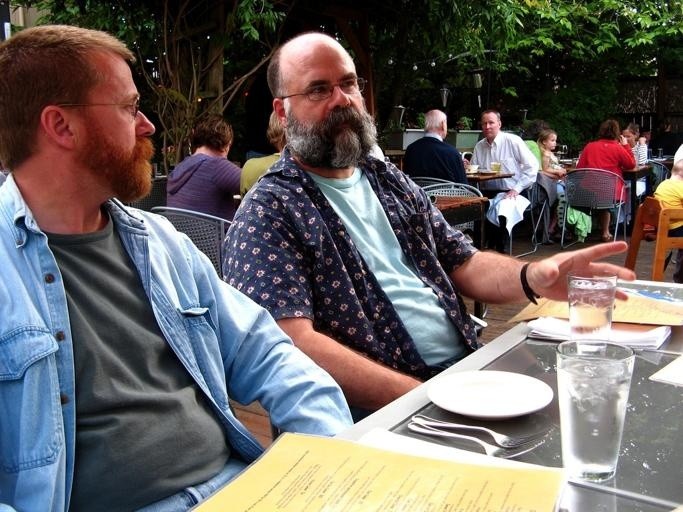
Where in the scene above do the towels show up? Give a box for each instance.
[485,192,531,236]
[526,317,671,353]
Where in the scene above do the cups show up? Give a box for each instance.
[555,339,636,482]
[566,268,618,355]
[491,161,501,173]
[656,148,663,159]
[647,149,653,160]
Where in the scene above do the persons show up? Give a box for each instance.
[401,110,468,196]
[654,159,683,284]
[167,113,245,279]
[220,32,636,423]
[577,120,636,243]
[654,120,678,158]
[674,143,683,164]
[620,123,647,236]
[642,130,651,143]
[0,24,353,511]
[536,129,574,244]
[471,111,539,253]
[240,109,286,201]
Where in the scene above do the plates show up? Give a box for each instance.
[560,159,573,165]
[429,369,554,420]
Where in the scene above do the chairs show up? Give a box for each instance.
[500,178,537,256]
[560,169,630,248]
[643,161,670,195]
[422,185,484,233]
[624,197,683,282]
[410,175,454,188]
[127,178,167,210]
[151,207,236,286]
[529,178,558,245]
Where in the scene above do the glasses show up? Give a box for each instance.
[58,97,139,118]
[282,77,369,100]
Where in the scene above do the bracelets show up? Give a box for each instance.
[520,262,542,306]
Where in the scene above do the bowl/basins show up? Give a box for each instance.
[466,164,480,170]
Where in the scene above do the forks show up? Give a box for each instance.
[411,413,554,448]
[407,420,545,459]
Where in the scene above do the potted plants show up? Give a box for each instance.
[402,113,427,151]
[501,125,520,137]
[447,115,483,149]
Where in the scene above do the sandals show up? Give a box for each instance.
[548,227,561,242]
[601,233,613,242]
[560,230,573,242]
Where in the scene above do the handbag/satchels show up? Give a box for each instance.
[570,189,594,216]
[648,160,664,191]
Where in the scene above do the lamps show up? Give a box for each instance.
[439,65,450,108]
[472,54,484,89]
